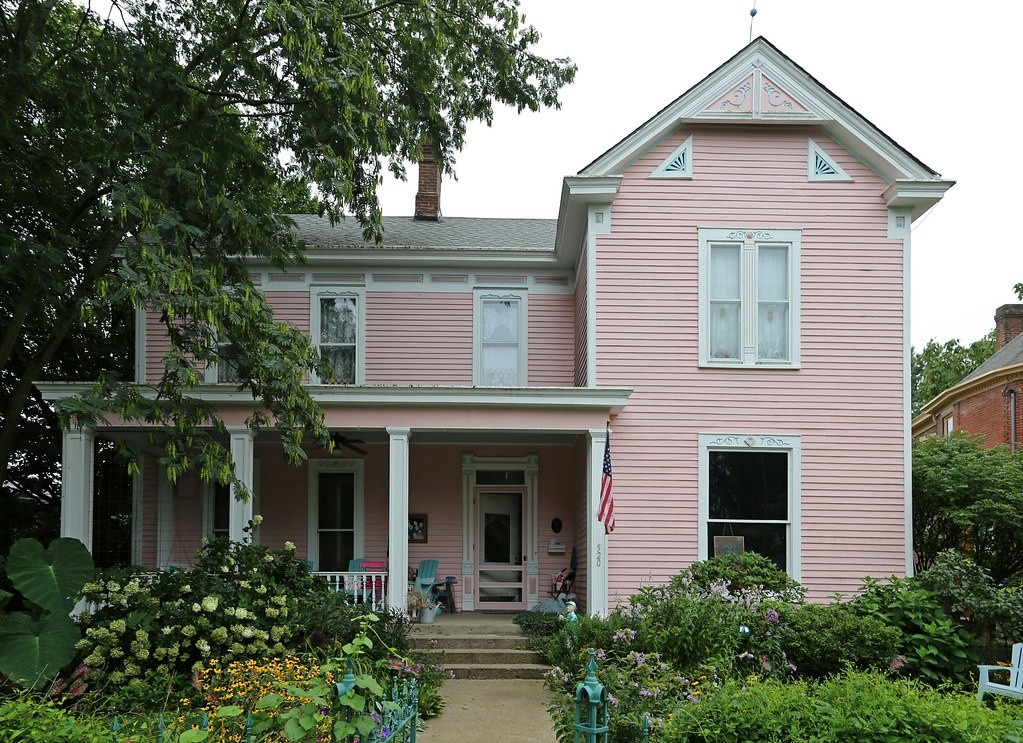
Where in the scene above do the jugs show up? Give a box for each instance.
[419,601,443,624]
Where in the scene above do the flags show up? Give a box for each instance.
[598,428,614,535]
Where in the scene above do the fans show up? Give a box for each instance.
[309,430,368,455]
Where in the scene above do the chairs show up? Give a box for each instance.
[548,572,577,600]
[337,559,372,604]
[977,642,1023,705]
[408,560,444,596]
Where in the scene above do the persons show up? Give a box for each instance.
[559,601,578,648]
